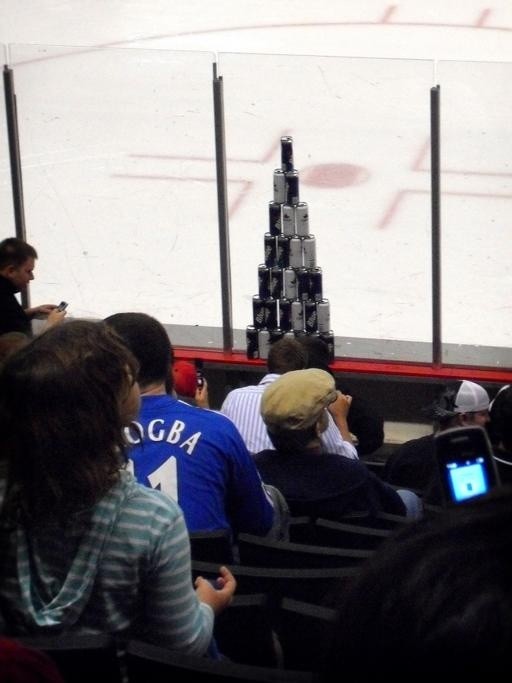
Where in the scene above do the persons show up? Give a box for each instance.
[102,311,295,549]
[2,235,66,339]
[1,317,240,661]
[4,333,510,523]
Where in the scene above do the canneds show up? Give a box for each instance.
[246,135,335,362]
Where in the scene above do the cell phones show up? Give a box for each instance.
[57,301,68,312]
[197,370,203,387]
[432,425,504,511]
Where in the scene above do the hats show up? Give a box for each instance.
[438,380,490,413]
[171,362,198,397]
[260,369,336,430]
